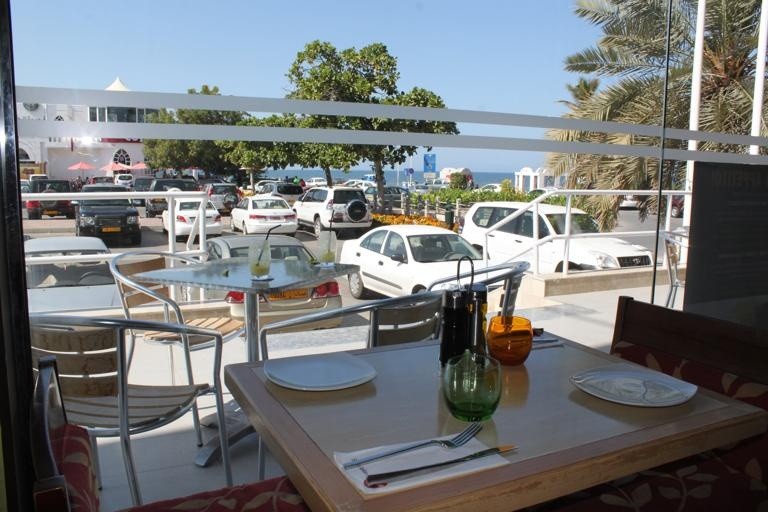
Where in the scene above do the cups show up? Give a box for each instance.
[246,239,273,281]
[443,355,503,423]
[488,314,533,367]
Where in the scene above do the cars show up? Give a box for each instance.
[132,176,155,205]
[145,179,185,218]
[482,184,502,193]
[204,183,236,212]
[362,174,386,184]
[228,196,298,236]
[525,187,559,196]
[335,180,365,186]
[162,199,221,241]
[261,184,304,207]
[240,180,284,191]
[30,174,49,183]
[179,177,199,190]
[305,177,328,187]
[409,184,429,194]
[21,180,32,206]
[365,186,411,207]
[25,236,122,312]
[425,179,448,190]
[341,224,494,299]
[351,180,374,192]
[115,174,133,187]
[198,179,229,189]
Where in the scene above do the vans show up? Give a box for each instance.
[461,201,657,274]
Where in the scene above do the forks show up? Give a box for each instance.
[342,424,483,469]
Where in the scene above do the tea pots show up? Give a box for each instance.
[439,255,487,369]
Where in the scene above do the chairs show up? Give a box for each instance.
[257,286,447,483]
[30,355,309,512]
[425,260,530,316]
[110,251,247,448]
[516,296,768,512]
[29,315,234,507]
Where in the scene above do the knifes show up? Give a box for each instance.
[366,444,519,485]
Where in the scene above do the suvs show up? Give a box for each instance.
[294,187,373,238]
[72,183,142,245]
[25,178,74,218]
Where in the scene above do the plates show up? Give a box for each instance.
[263,352,377,392]
[570,367,699,408]
